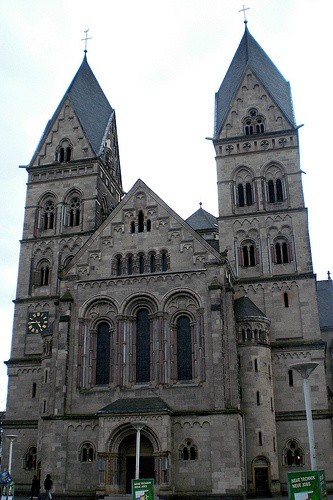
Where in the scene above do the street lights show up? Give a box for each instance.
[5,435,19,475]
[130,419,147,479]
[289,359,320,470]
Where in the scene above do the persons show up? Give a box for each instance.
[44,474,53,499]
[30,475,41,498]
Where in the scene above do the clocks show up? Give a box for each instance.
[26,312,48,334]
